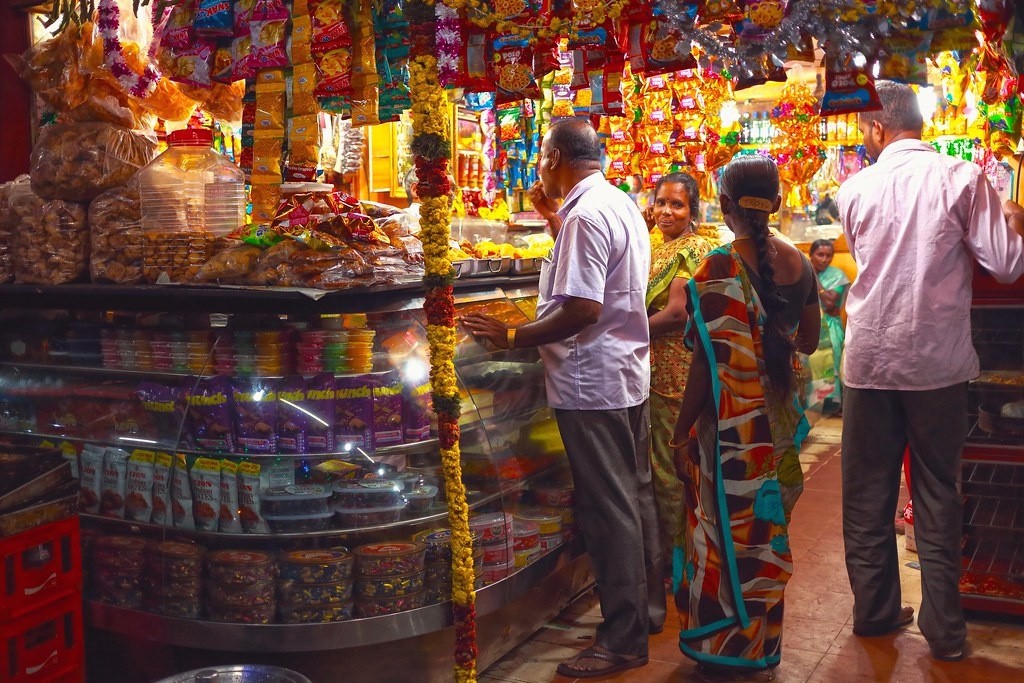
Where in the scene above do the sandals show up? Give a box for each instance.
[929,641,967,661]
[850,606,915,636]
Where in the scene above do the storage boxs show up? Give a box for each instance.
[0,515,85,683]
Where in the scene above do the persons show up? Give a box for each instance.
[646,156,851,672]
[459,116,668,679]
[835,79,1023,661]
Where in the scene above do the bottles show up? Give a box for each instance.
[821,113,864,141]
[922,96,967,136]
[134,127,245,285]
[458,155,486,188]
[738,106,780,144]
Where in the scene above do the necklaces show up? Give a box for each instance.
[735,233,774,241]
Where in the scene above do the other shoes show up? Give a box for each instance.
[690,659,777,683]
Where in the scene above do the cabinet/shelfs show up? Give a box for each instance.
[957,282,1024,615]
[0,276,596,683]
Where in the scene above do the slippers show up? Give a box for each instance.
[646,623,665,636]
[555,643,651,679]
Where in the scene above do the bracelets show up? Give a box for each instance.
[818,289,824,295]
[507,328,516,349]
